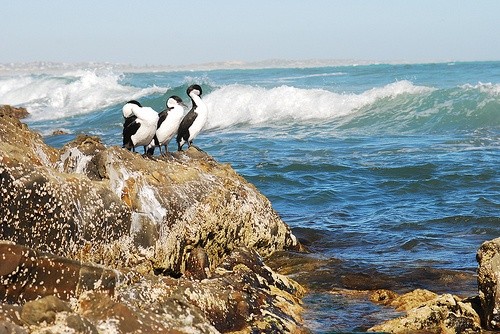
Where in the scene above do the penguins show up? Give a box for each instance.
[122,100,159,159]
[144,95,189,162]
[175,84,208,153]
